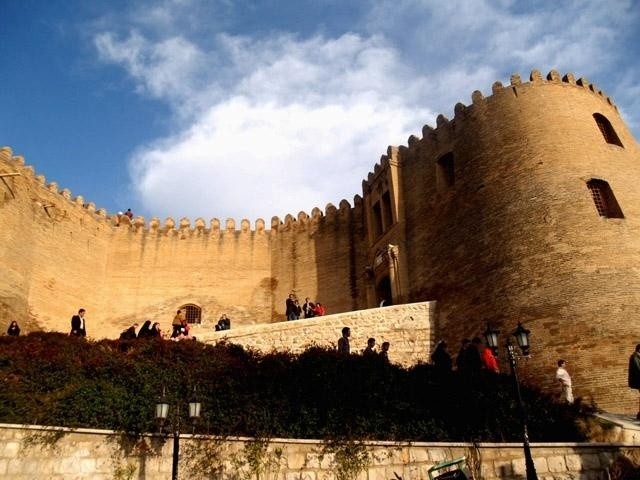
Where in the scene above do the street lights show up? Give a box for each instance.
[154,383,202,480]
[481,320,538,480]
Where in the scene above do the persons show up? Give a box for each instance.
[7,320,20,336]
[285,294,325,320]
[375,294,388,308]
[214,312,231,331]
[70,308,93,339]
[337,327,351,354]
[168,309,190,338]
[118,320,163,339]
[430,340,452,372]
[628,345,640,418]
[555,359,576,403]
[362,338,391,361]
[457,337,500,373]
[112,207,133,226]
